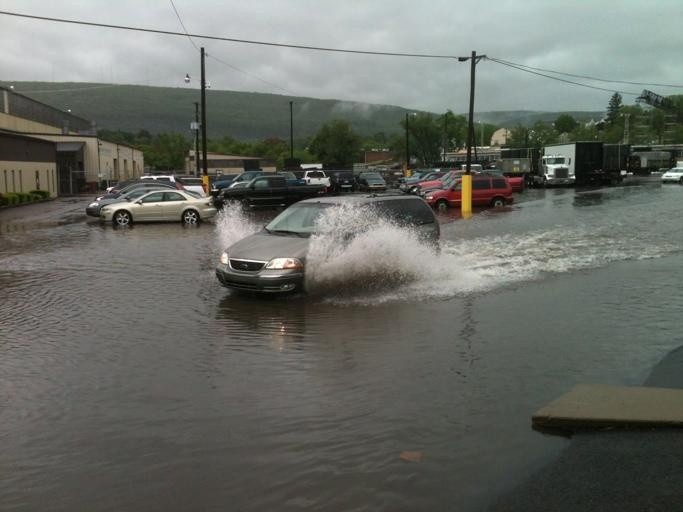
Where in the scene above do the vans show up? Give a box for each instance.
[213,191,441,296]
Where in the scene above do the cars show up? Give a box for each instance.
[660,167,683,183]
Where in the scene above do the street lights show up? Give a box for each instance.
[182,73,208,179]
[404,109,418,171]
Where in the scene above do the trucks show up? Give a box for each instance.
[626,150,679,172]
[526,153,575,189]
